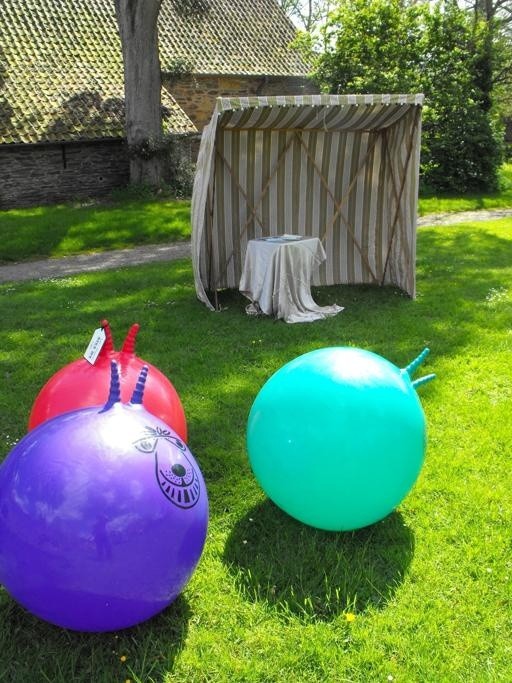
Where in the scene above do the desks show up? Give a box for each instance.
[239,234,345,324]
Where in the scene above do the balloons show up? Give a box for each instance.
[245,346,439,532]
[0,359,209,633]
[27,319,187,445]
